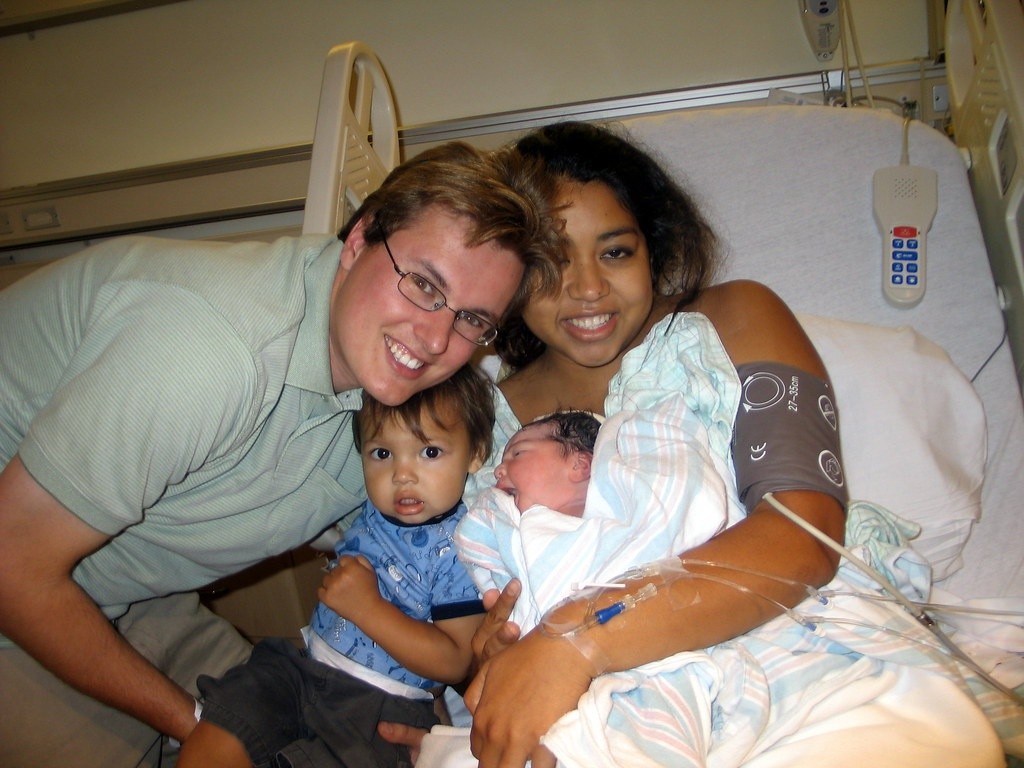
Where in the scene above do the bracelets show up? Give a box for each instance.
[170,698,203,749]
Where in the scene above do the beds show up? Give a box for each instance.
[300,0,1024,768]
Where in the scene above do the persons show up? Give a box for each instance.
[464,120,1007,768]
[176,366,495,768]
[496,410,600,522]
[0,142,557,768]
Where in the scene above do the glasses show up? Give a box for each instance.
[372,213,501,347]
